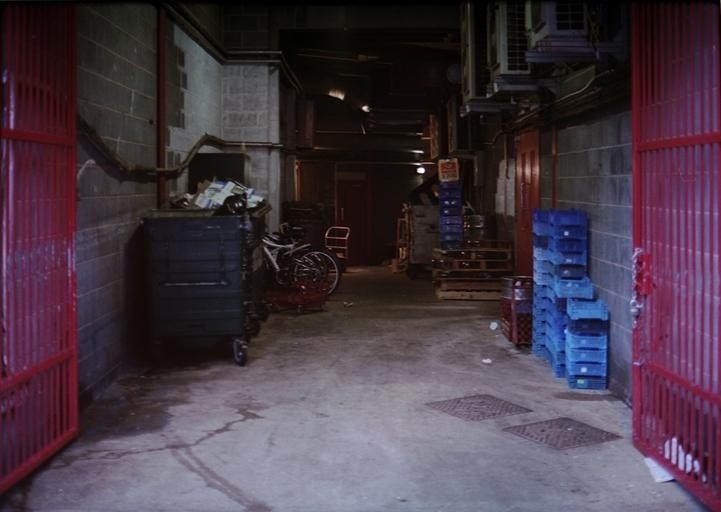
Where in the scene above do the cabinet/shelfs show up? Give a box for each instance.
[188,153,251,194]
[431,240,513,301]
[501,276,533,347]
[396,218,409,270]
[323,226,352,261]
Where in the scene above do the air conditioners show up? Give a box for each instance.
[522,0,618,50]
[458,0,513,106]
[485,0,544,77]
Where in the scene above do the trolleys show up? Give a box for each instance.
[323,227,350,272]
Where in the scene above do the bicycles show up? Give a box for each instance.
[254,227,341,300]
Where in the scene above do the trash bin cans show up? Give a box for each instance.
[136,196,272,368]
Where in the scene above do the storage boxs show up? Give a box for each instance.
[526,208,608,389]
